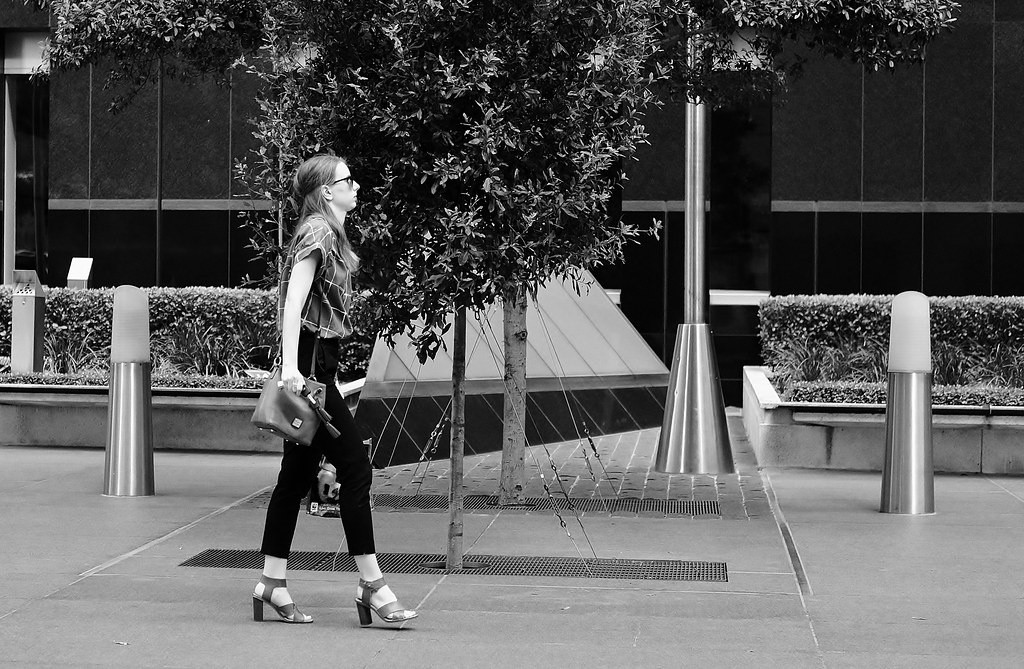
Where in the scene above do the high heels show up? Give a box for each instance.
[355,580,419,626]
[252,575,314,623]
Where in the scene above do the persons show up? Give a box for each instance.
[253,153,418,627]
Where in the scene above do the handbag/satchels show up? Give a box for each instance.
[250,363,327,445]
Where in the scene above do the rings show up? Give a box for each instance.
[293,383,297,387]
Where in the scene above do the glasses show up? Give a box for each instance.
[329,175,354,188]
